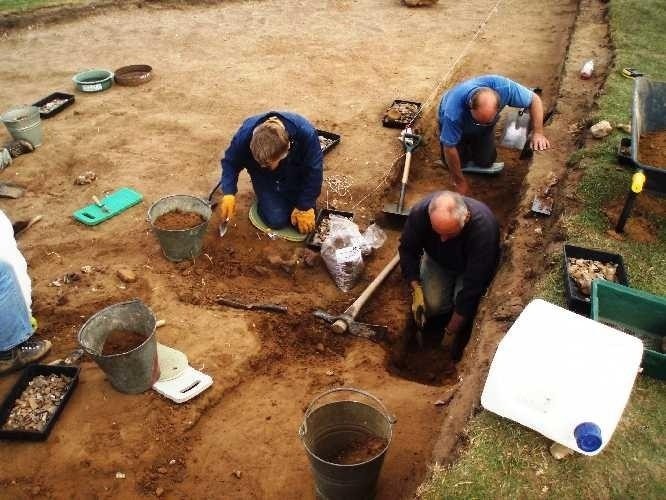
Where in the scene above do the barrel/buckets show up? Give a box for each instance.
[480,298,644,458]
[77,297,161,393]
[0,105,41,148]
[298,387,397,500]
[145,194,213,262]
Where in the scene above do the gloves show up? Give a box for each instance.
[291,207,316,233]
[441,328,456,352]
[220,194,237,222]
[411,287,425,324]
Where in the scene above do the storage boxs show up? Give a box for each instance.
[0,364,80,440]
[32,92,74,118]
[563,244,666,382]
[306,207,355,251]
[317,130,340,154]
[382,99,422,128]
[617,138,637,168]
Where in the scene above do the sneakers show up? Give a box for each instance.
[0,339,53,376]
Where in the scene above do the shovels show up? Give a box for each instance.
[217,218,229,238]
[382,133,424,218]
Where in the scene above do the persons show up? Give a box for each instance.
[0,260,52,375]
[437,74,552,198]
[219,111,324,234]
[394,189,502,353]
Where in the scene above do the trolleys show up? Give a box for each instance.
[615,77,666,232]
